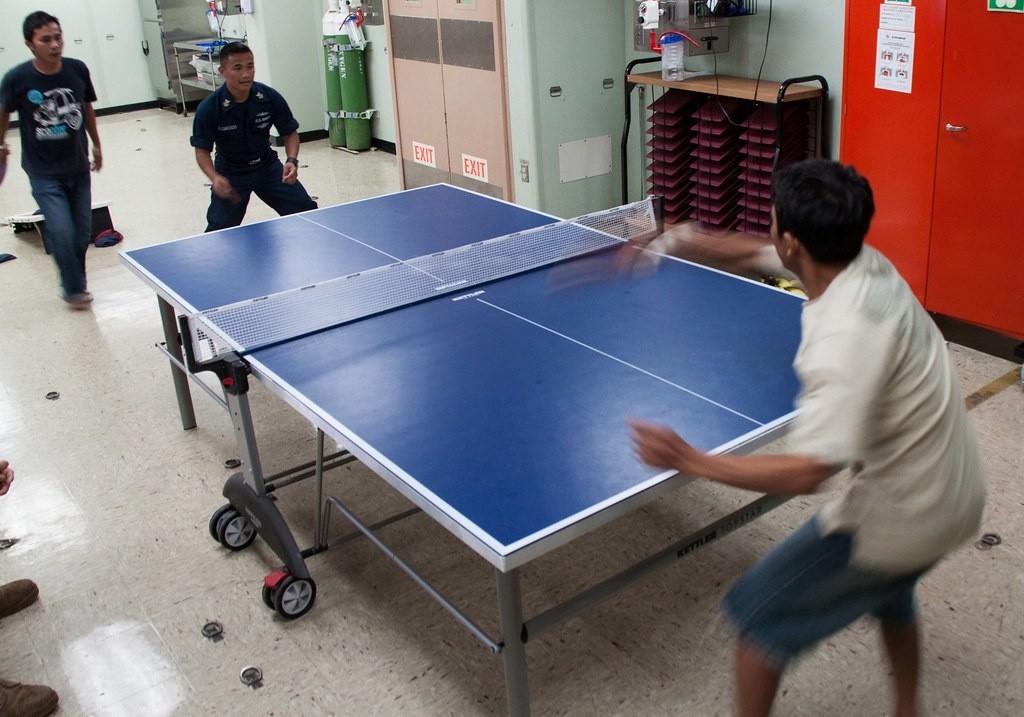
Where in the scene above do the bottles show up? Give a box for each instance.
[660,33,686,82]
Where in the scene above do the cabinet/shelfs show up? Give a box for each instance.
[138,0,218,115]
[381,0,643,222]
[840,0,1024,345]
[173,37,247,118]
[620,57,829,244]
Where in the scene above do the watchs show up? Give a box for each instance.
[284,157,299,168]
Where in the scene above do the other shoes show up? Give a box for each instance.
[63,291,93,306]
[0,578,40,619]
[0,677,59,717]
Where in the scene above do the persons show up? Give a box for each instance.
[0,10,103,309]
[188,41,318,235]
[615,156,988,715]
